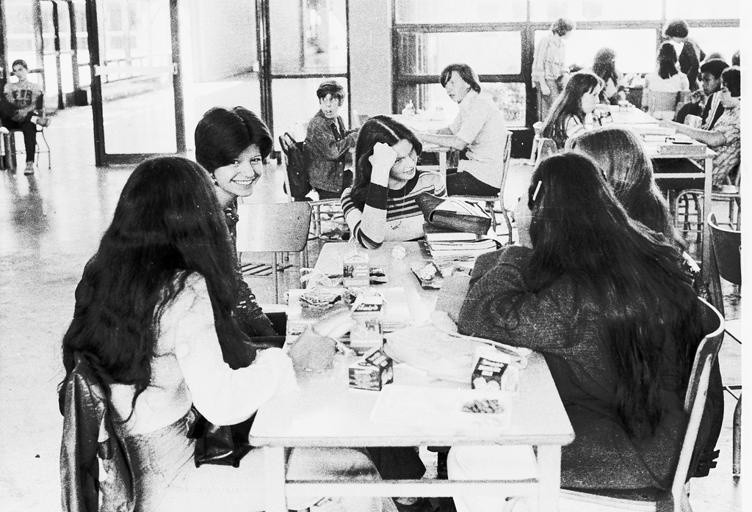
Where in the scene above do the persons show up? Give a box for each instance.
[674,59,728,131]
[304,82,361,201]
[570,129,723,477]
[417,153,710,512]
[653,66,740,191]
[532,14,577,120]
[533,74,605,160]
[594,47,621,104]
[413,66,502,196]
[655,21,699,93]
[57,157,398,512]
[196,108,440,512]
[341,118,448,255]
[644,44,691,121]
[0,59,51,177]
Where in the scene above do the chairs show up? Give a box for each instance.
[278,132,353,239]
[63,356,136,512]
[561,296,726,511]
[533,121,546,139]
[6,68,52,171]
[233,198,312,304]
[538,139,557,164]
[709,213,740,488]
[675,167,740,245]
[682,195,705,243]
[450,131,516,245]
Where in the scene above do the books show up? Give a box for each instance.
[422,224,504,278]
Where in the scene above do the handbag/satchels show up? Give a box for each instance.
[278,132,313,199]
[415,191,492,235]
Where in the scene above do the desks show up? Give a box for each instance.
[249,241,576,449]
[367,113,453,153]
[580,100,718,159]
[611,73,645,101]
